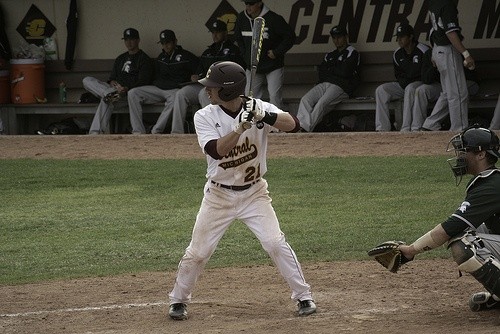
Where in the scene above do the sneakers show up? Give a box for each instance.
[296,299,317,316]
[169,303,188,321]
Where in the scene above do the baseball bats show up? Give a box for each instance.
[244,17,266,122]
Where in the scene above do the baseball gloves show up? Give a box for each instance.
[369,242,414,275]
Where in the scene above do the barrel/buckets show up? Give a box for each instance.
[9,58,46,105]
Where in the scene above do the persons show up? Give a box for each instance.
[168,61,316,321]
[127,29,200,134]
[420,1,475,136]
[374,24,477,133]
[293,25,368,133]
[169,20,245,133]
[233,0,294,109]
[81,28,156,133]
[396,127,500,312]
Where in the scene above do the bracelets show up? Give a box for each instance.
[462,50,471,58]
[412,234,435,254]
[262,111,277,126]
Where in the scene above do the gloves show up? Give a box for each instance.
[241,95,266,122]
[234,111,254,136]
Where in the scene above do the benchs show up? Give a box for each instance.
[0,47,500,134]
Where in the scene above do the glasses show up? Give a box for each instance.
[245,1,257,5]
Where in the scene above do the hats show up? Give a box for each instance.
[330,26,346,37]
[121,28,139,39]
[156,30,177,44]
[393,24,415,37]
[208,20,227,33]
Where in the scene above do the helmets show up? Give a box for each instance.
[198,62,248,101]
[445,125,500,157]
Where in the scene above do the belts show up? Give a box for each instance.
[212,180,259,191]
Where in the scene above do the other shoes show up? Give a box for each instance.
[471,294,500,312]
[103,93,121,105]
